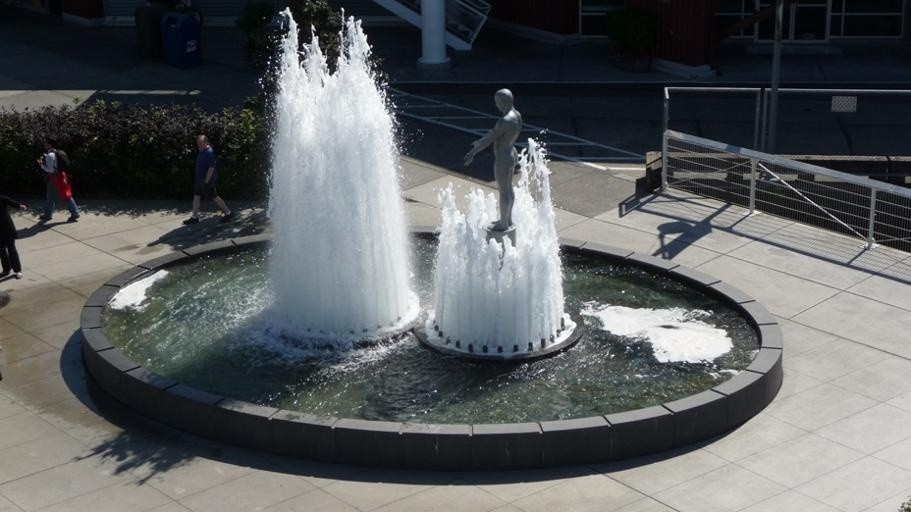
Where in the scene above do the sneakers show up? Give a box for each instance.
[69,214,79,221]
[16,272,23,279]
[183,217,199,224]
[0,271,9,277]
[220,211,233,222]
[40,215,50,219]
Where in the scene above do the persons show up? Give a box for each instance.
[36,134,81,222]
[0,194,28,281]
[462,87,523,231]
[183,133,234,226]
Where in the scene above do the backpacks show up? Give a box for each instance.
[47,149,71,172]
